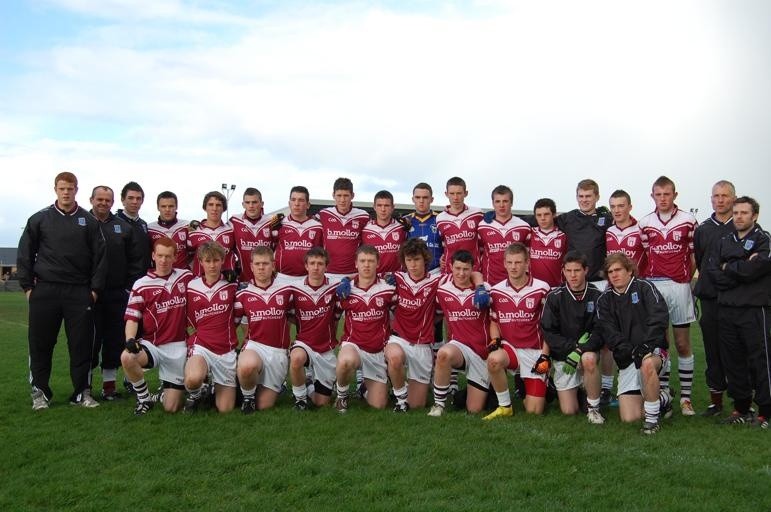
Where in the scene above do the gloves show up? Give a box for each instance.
[125,338,142,354]
[484,211,495,223]
[189,220,200,230]
[562,349,582,374]
[395,216,412,231]
[335,279,350,300]
[488,337,503,351]
[531,354,552,375]
[473,284,491,310]
[634,344,653,369]
[270,213,284,230]
[222,269,237,283]
[312,211,321,220]
[386,276,396,286]
[576,329,592,346]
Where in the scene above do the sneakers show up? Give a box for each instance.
[577,386,611,425]
[336,394,348,413]
[70,389,100,408]
[720,406,769,428]
[679,399,696,416]
[428,402,446,416]
[545,377,556,404]
[101,376,255,416]
[391,392,408,414]
[641,387,677,435]
[513,372,526,400]
[700,403,722,416]
[481,405,513,421]
[351,382,368,400]
[292,377,313,410]
[31,391,49,410]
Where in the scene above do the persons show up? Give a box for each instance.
[16,171,421,415]
[333,176,771,436]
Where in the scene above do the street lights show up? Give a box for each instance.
[219,180,236,219]
[689,205,700,219]
